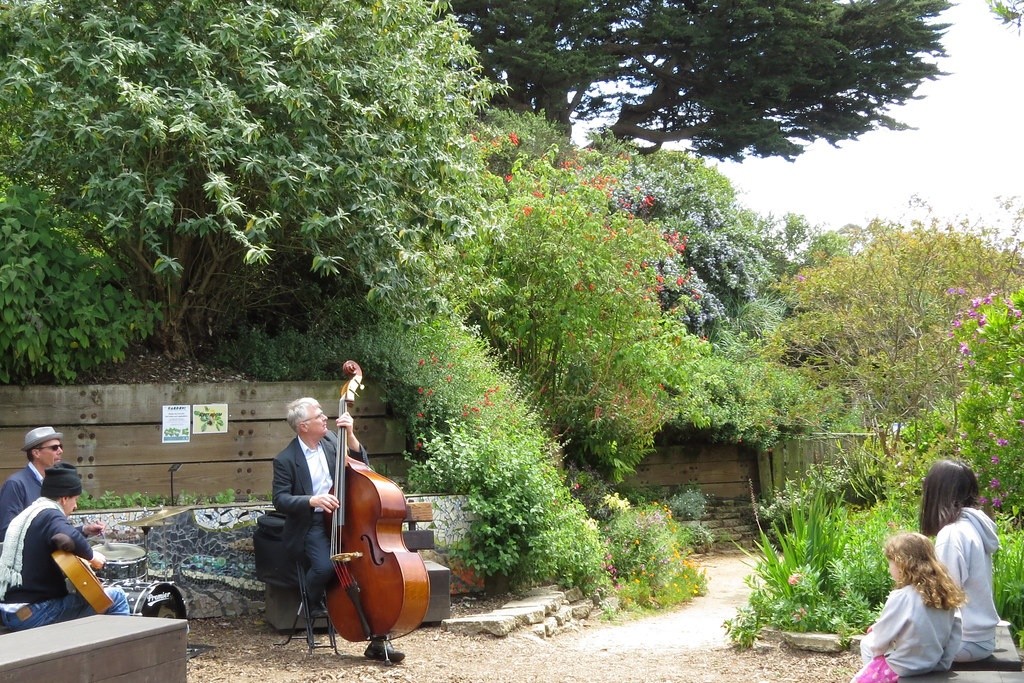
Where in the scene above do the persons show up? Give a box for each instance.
[0,426,131,632]
[850,533,965,683]
[272,397,406,663]
[920,460,1002,662]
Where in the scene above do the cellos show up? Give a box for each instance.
[325,360,430,666]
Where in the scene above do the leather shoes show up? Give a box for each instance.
[309,600,328,618]
[364,643,404,662]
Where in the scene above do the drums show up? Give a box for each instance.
[99,579,187,620]
[91,543,149,583]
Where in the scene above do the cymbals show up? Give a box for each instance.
[136,505,194,525]
[118,519,175,527]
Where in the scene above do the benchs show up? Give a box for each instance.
[0,614,187,683]
[264,502,450,630]
[897,621,1024,683]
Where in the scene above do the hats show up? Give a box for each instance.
[41,463,82,498]
[21,426,63,452]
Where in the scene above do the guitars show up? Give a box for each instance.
[52,549,114,614]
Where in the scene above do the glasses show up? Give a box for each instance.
[301,411,324,423]
[33,443,63,451]
